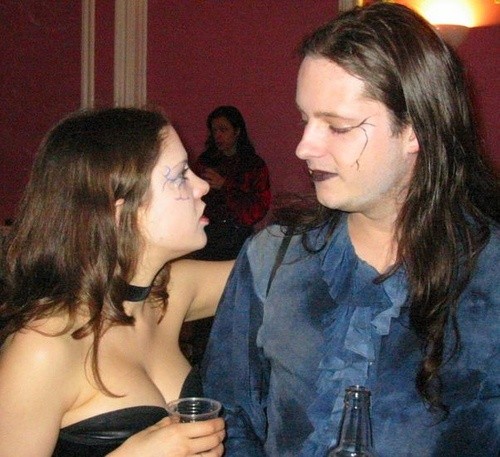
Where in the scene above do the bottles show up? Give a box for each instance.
[321,383,376,457]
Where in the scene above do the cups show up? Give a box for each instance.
[164,397,222,425]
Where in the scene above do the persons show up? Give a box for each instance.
[189,103,273,266]
[197,2,499,456]
[0,104,237,457]
